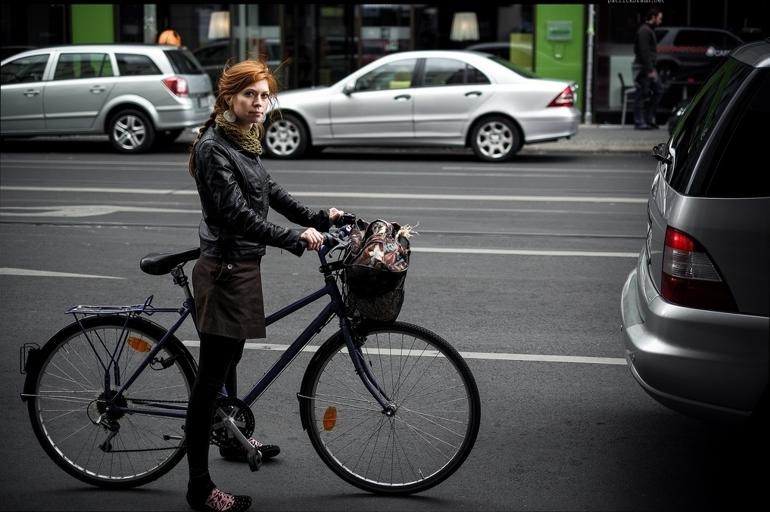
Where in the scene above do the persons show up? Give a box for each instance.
[632,10,663,130]
[186,58,344,512]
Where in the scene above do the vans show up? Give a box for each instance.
[621,41,770,434]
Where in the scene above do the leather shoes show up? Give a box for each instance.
[184,488,252,512]
[636,120,659,130]
[225,441,281,462]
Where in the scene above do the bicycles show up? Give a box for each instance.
[17,214,480,497]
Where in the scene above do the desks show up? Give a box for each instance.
[664,78,702,102]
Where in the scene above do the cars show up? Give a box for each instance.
[466,43,582,82]
[253,50,581,162]
[654,27,747,80]
[0,44,216,154]
[193,39,282,95]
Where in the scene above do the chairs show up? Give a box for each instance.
[617,71,638,125]
